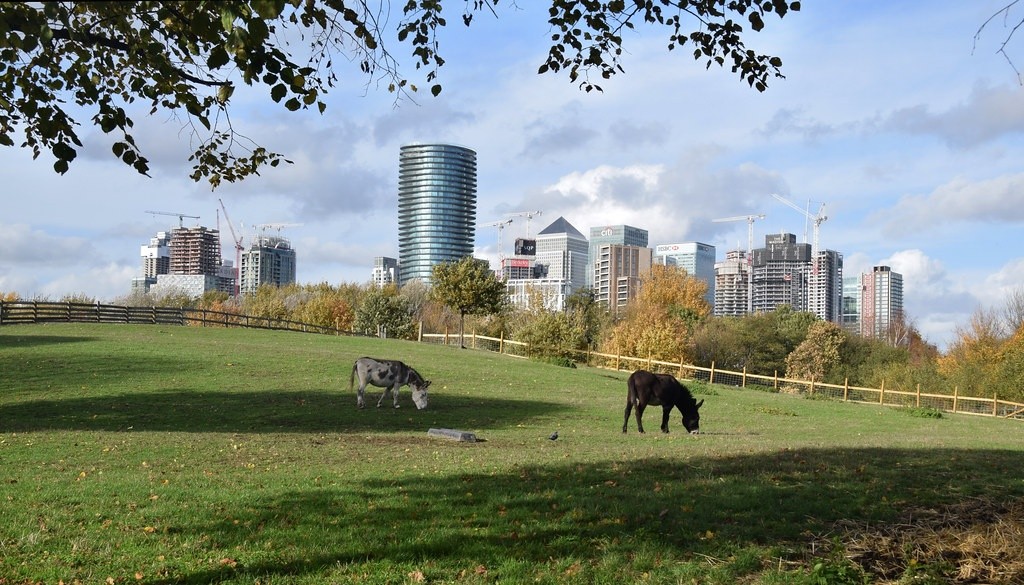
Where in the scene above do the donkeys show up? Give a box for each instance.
[347,357,432,410]
[622,369,704,434]
